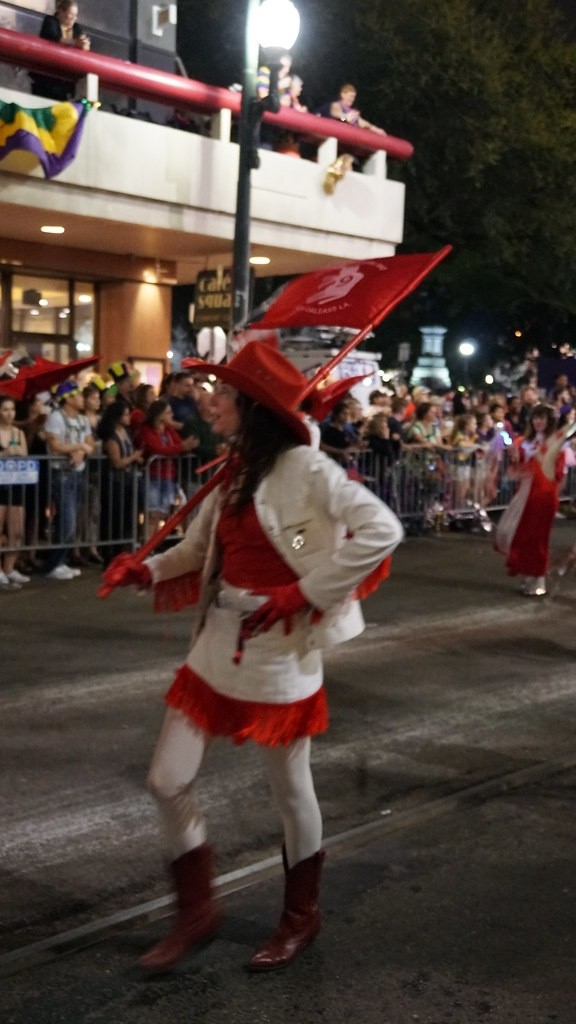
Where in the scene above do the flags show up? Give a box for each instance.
[250,245,451,330]
[0,348,102,400]
[303,371,374,423]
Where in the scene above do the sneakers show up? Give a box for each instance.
[87,553,105,565]
[0,568,31,584]
[44,565,82,581]
[70,555,88,566]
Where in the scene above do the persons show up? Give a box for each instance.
[0,375,222,586]
[493,405,566,595]
[230,47,387,172]
[103,340,403,969]
[298,374,576,539]
[28,0,91,103]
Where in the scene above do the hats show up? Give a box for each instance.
[108,362,130,383]
[54,380,78,402]
[181,341,312,447]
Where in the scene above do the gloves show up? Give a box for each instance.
[103,553,149,588]
[248,582,307,636]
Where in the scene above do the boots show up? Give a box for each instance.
[133,840,222,968]
[249,840,326,971]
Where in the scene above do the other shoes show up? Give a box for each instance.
[520,579,546,596]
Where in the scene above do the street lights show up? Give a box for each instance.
[457,341,476,386]
[227,0,299,334]
